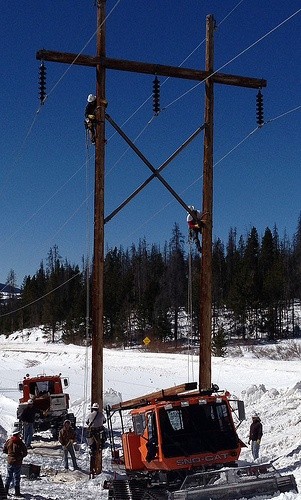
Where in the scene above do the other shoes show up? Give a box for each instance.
[26,445,32,448]
[24,443,27,447]
[198,247,202,253]
[91,139,95,145]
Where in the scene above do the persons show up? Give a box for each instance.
[86,402,108,444]
[21,399,43,449]
[59,419,81,470]
[186,205,206,252]
[248,413,263,460]
[3,430,28,496]
[84,92,109,144]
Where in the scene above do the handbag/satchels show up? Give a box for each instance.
[85,436,95,445]
[83,429,93,438]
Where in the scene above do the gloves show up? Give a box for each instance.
[194,224,200,228]
[88,114,95,120]
[256,439,261,444]
[248,439,251,445]
[100,99,107,104]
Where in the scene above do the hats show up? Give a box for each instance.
[63,420,71,427]
[28,399,33,404]
[11,427,21,435]
[252,412,260,417]
[92,403,99,409]
[186,205,194,213]
[87,94,96,102]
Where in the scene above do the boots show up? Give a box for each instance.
[4,484,12,497]
[13,485,25,497]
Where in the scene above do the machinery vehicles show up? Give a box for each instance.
[102,382,299,500]
[14,373,77,447]
